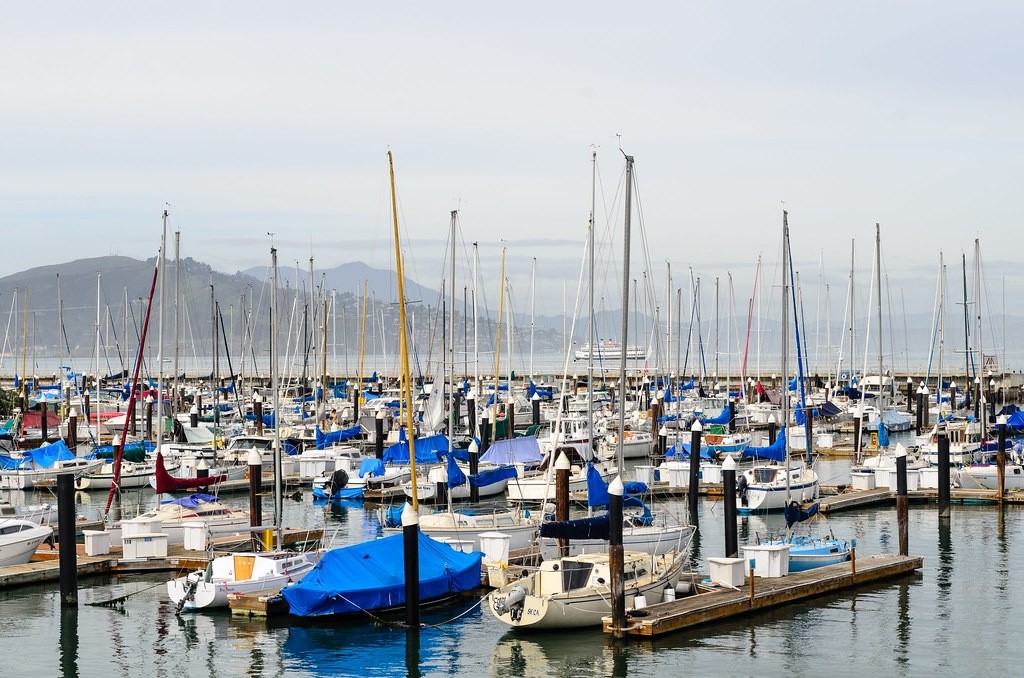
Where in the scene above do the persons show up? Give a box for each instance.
[412,412,421,436]
[330,409,337,425]
[386,414,394,430]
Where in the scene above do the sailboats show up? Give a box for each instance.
[2,133,1024,637]
